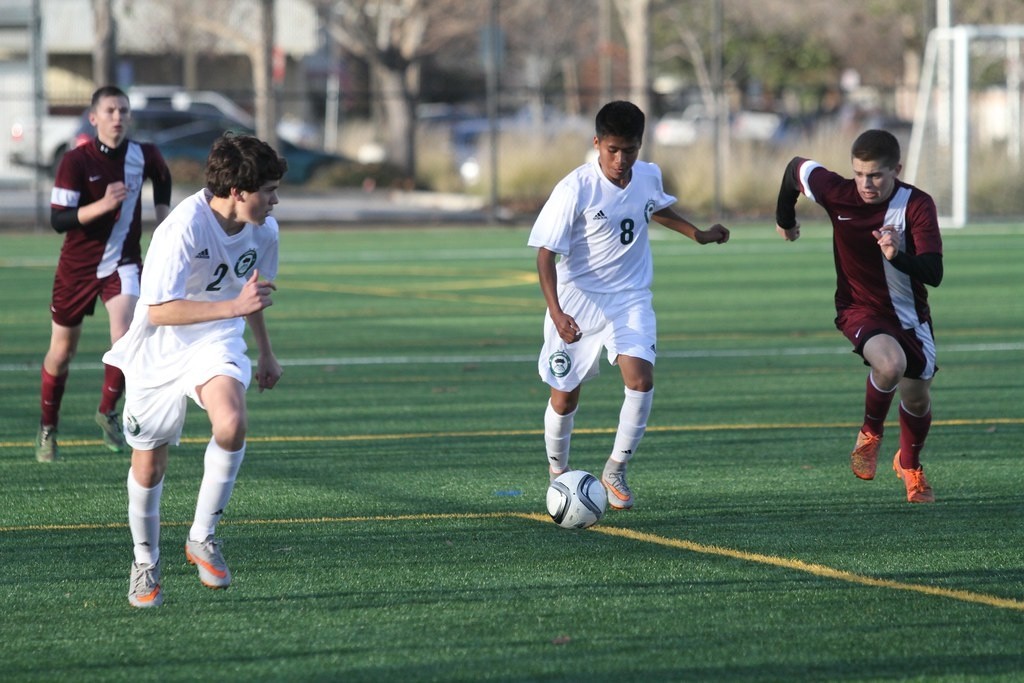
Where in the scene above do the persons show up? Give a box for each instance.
[102,132,288,607]
[33,84,172,462]
[776,129,943,502]
[526,101,729,509]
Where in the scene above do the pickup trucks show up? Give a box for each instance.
[10,84,255,177]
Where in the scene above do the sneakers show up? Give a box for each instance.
[36,424,57,463]
[851,430,882,480]
[94,406,125,452]
[893,447,935,503]
[549,465,570,484]
[128,558,163,607]
[184,534,232,590]
[602,458,633,509]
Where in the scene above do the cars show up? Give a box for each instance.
[71,106,342,185]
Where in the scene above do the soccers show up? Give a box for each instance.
[546,470,608,530]
